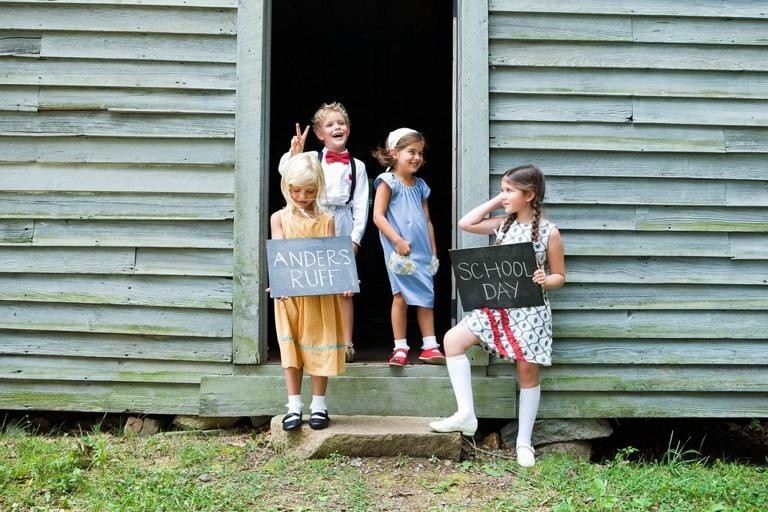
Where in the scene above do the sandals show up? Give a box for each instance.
[418,347,446,364]
[388,348,408,367]
[309,410,331,430]
[281,411,302,431]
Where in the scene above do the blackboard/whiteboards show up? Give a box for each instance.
[449,242,545,312]
[266,235,361,298]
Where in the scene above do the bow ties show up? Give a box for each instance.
[325,150,351,164]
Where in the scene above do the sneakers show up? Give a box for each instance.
[429,416,478,437]
[515,445,537,467]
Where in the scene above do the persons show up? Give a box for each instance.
[265,102,369,431]
[373,128,446,367]
[428,165,565,468]
